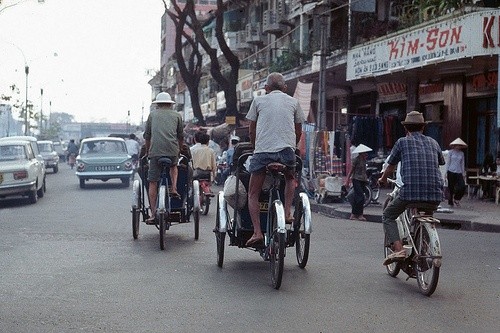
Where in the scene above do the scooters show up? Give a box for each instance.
[188,159,215,216]
[65,149,76,170]
[377,176,442,296]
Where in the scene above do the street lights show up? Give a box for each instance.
[6,39,67,142]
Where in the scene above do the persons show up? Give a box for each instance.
[143,92,184,221]
[483,156,500,200]
[190,132,216,181]
[344,144,372,220]
[446,138,468,208]
[245,72,304,246]
[67,140,78,162]
[87,143,96,152]
[379,111,445,266]
[125,134,140,161]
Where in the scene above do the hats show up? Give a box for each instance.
[401,111,432,126]
[152,91,176,104]
[352,144,373,153]
[449,137,467,148]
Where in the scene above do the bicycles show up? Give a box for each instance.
[131,156,138,181]
[362,172,381,209]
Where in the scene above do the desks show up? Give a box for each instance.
[480,175,500,202]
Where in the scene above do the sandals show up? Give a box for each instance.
[383,250,406,266]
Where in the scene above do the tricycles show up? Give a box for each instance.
[212,142,312,291]
[130,141,203,250]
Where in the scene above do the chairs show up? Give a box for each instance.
[465,168,482,200]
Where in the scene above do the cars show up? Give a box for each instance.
[0,140,43,204]
[36,140,60,174]
[0,136,47,193]
[53,142,68,162]
[74,137,133,189]
